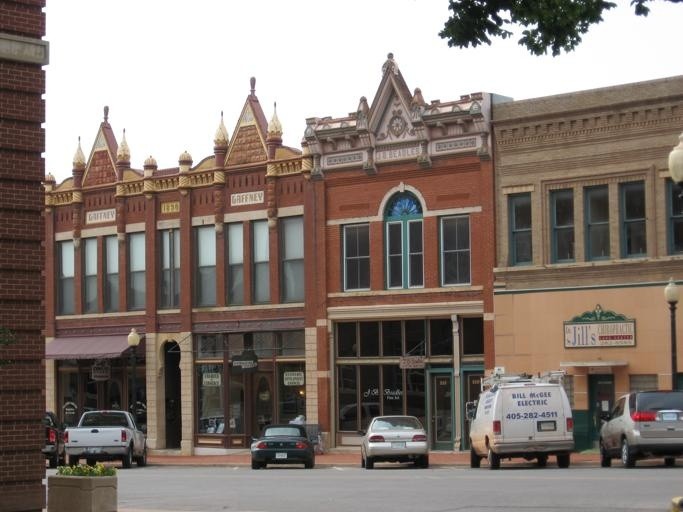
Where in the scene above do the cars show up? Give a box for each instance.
[339,402,381,432]
[250,424,315,469]
[361,415,428,469]
[598,391,683,468]
[127,327,140,417]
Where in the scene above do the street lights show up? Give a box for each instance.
[667,132,683,207]
[665,275,680,390]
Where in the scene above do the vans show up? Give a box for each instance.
[468,369,575,470]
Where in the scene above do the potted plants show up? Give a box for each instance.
[47,463,118,512]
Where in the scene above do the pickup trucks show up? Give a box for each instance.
[45,410,146,469]
